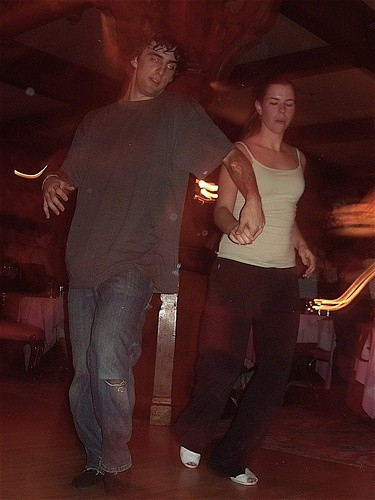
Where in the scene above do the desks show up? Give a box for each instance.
[295,315,334,384]
[7,292,64,372]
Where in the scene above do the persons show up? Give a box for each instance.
[22,226,56,291]
[0,257,29,292]
[6,221,37,292]
[178,75,317,487]
[177,236,373,422]
[40,25,266,498]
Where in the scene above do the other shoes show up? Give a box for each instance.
[230,469,258,486]
[72,469,104,491]
[180,445,201,469]
[103,467,132,495]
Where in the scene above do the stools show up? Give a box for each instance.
[0,322,46,374]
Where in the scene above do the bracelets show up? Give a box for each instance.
[41,174,59,191]
